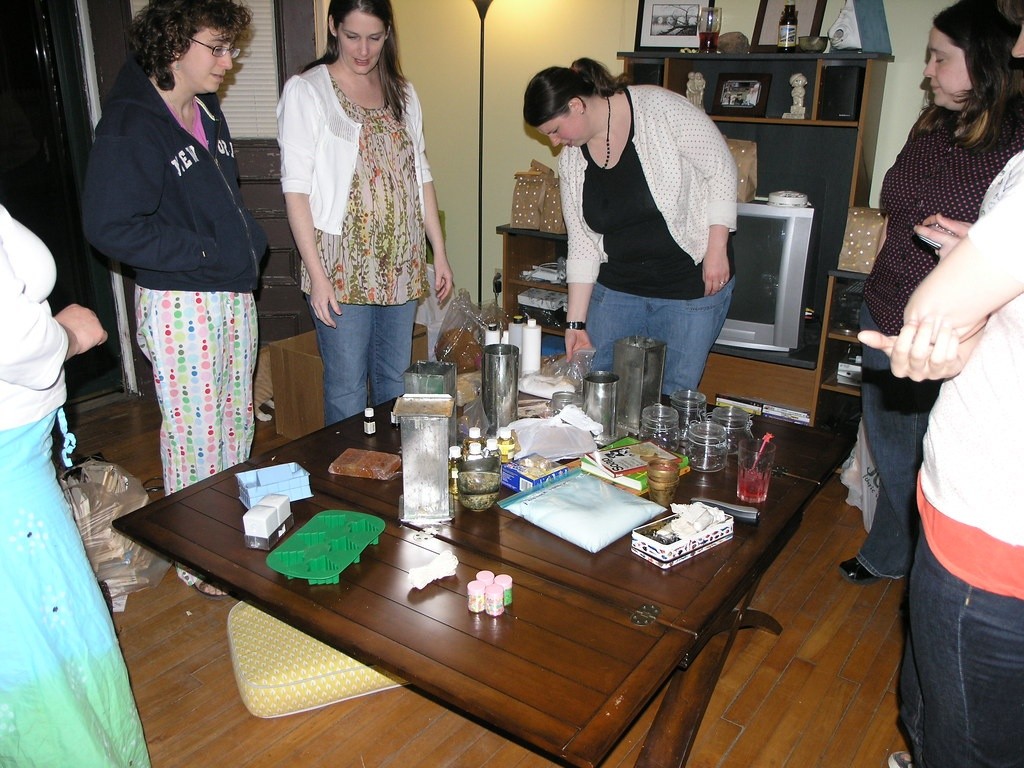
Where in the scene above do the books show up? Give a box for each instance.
[580,436,690,497]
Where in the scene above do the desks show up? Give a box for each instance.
[111,385,857,768]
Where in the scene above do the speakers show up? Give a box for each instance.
[637,62,664,87]
[819,65,866,121]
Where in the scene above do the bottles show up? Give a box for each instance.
[522,319,542,377]
[466,570,513,617]
[363,408,377,437]
[508,316,527,378]
[484,322,501,346]
[777,0,798,54]
[448,426,515,500]
[638,389,753,473]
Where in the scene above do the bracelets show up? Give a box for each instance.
[566,321,588,331]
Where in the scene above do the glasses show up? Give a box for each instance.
[186,33,240,59]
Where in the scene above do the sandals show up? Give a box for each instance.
[192,576,229,600]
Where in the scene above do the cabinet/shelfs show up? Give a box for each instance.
[616,48,895,423]
[814,270,894,431]
[496,221,572,359]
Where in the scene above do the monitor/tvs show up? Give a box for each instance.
[713,201,815,351]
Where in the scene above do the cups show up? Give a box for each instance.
[552,392,578,423]
[647,478,679,520]
[582,371,620,441]
[698,6,723,54]
[736,438,776,504]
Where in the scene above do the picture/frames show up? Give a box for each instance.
[749,0,828,51]
[712,72,772,117]
[634,0,716,51]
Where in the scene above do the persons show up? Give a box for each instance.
[276,0,455,430]
[837,0,1023,588]
[82,1,273,601]
[856,25,1024,767]
[686,71,706,110]
[0,202,150,768]
[523,56,738,405]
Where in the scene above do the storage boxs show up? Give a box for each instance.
[501,452,567,492]
[267,323,427,441]
[631,503,733,568]
[234,462,315,508]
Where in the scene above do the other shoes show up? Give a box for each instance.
[888,751,913,768]
[838,555,883,583]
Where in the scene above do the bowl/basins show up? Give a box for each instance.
[455,457,501,512]
[798,35,829,54]
[646,459,678,483]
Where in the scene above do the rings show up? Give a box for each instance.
[720,281,726,285]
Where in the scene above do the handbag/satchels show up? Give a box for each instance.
[58,460,174,600]
[841,417,885,533]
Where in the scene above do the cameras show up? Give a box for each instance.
[919,222,956,248]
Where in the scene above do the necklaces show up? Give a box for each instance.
[598,96,610,172]
